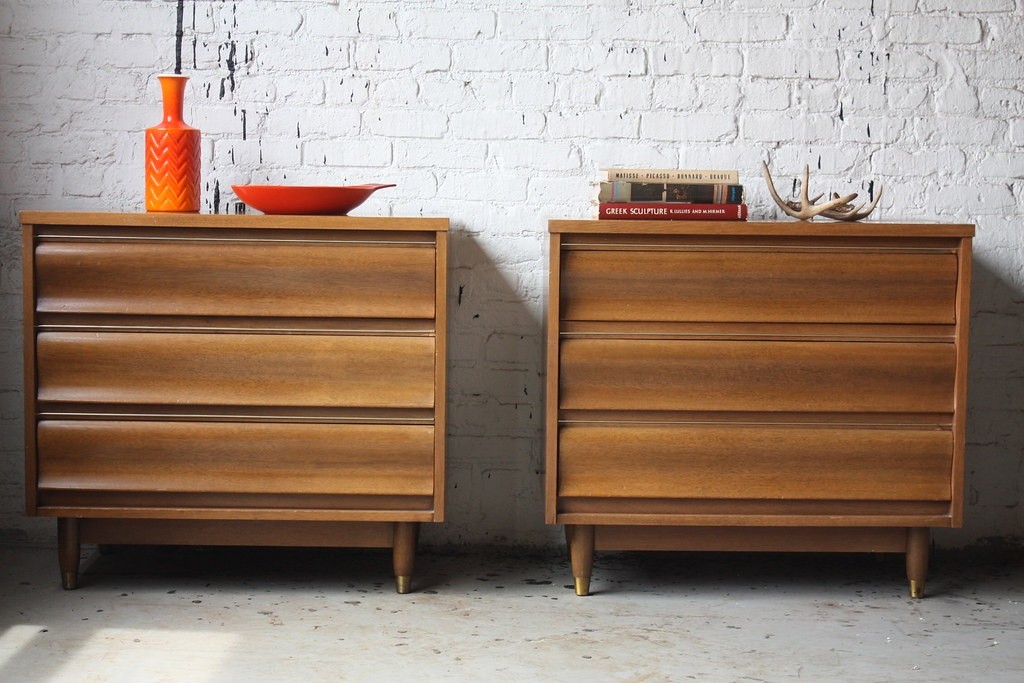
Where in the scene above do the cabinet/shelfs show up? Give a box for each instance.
[18,210,451,595]
[544,219,976,598]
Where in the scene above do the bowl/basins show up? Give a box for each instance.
[233,185,398,216]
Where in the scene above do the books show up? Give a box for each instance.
[598,168,748,220]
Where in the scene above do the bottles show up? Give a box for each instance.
[146,75,201,212]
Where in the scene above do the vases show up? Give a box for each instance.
[145,74,201,213]
